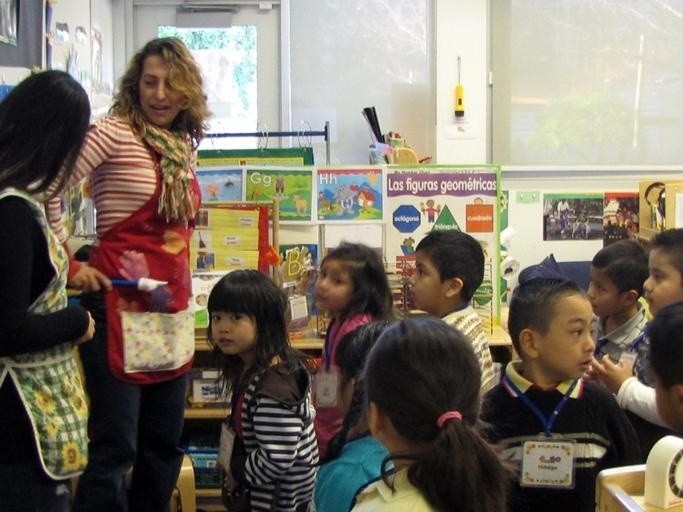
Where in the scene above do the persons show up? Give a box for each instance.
[643,300,683,433]
[582,227,683,431]
[586,239,650,434]
[407,229,496,416]
[351,318,523,512]
[0,69,97,512]
[206,269,320,512]
[312,319,395,512]
[42,36,214,512]
[303,240,394,466]
[480,253,644,512]
